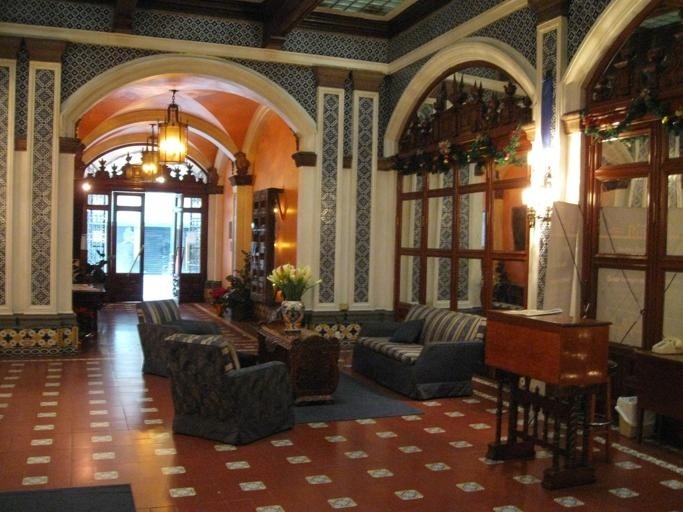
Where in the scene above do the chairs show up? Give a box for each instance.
[135,299,221,377]
[162,333,295,444]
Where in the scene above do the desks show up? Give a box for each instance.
[633,349,681,446]
[70,282,106,338]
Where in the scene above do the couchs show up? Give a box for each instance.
[351,305,487,401]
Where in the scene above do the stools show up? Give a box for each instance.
[583,360,619,464]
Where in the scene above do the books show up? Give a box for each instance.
[501,308,565,318]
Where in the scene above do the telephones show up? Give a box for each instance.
[651,337,682,355]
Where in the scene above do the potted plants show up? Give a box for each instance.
[221,249,253,321]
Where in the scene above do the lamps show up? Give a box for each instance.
[156,87,190,164]
[524,206,550,228]
[121,121,164,186]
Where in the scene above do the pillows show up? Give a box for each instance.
[378,319,400,336]
[234,351,259,368]
[388,318,425,343]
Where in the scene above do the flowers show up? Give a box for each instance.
[266,262,322,300]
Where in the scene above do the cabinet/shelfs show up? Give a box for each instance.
[249,188,283,301]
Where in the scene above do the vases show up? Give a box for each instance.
[281,300,305,335]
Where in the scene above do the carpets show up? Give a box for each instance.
[295,371,424,423]
[0,482,136,512]
